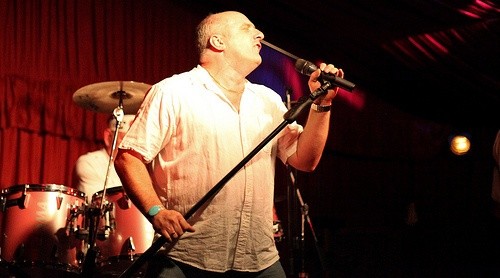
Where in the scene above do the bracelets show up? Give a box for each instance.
[148,204,165,221]
[311,103,331,112]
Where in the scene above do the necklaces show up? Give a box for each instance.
[208,72,244,93]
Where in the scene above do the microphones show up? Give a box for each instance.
[295,59,355,93]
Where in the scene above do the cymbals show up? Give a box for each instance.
[72,80,155,114]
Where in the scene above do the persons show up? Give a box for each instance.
[114,11,344,278]
[72,115,138,248]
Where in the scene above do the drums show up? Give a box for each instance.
[91,186,165,268]
[0,183,87,278]
[273,202,287,241]
